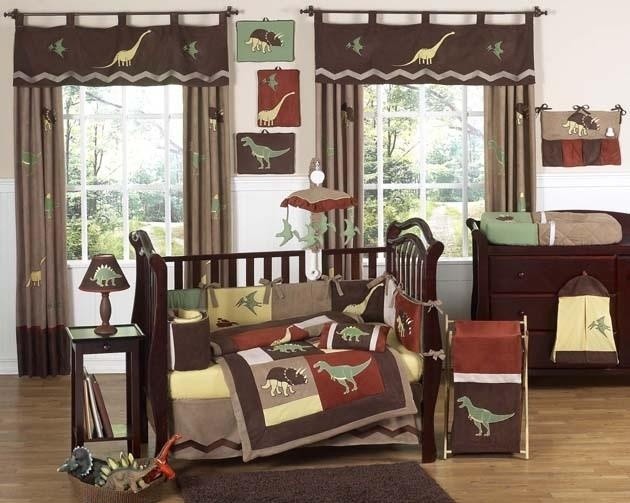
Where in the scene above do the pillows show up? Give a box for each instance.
[320,320,393,352]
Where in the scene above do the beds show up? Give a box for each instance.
[128,217,444,465]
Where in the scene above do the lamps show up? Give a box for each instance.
[76,252,131,335]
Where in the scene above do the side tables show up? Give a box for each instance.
[64,321,147,471]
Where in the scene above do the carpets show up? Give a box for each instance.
[176,459,451,502]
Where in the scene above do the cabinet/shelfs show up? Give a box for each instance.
[466,208,629,386]
